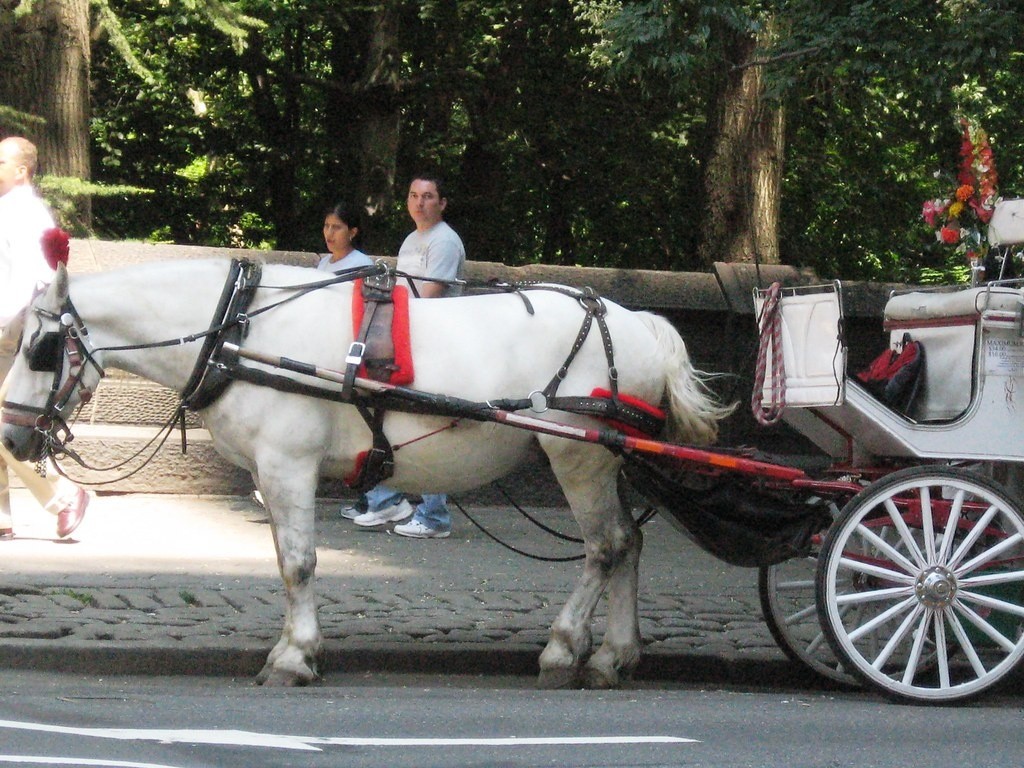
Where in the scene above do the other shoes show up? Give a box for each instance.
[251,490,265,509]
[340,506,361,519]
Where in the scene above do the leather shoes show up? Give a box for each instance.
[0,527,12,537]
[56,486,90,538]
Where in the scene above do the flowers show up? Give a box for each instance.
[920,80,1004,291]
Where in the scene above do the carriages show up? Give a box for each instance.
[1,195,1024,705]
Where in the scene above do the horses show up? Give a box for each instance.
[2,265,742,692]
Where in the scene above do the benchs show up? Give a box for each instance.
[882,197,1023,422]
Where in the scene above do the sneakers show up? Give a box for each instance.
[393,520,451,538]
[352,498,413,526]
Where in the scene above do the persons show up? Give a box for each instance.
[0,137,90,538]
[352,172,467,538]
[248,202,374,519]
